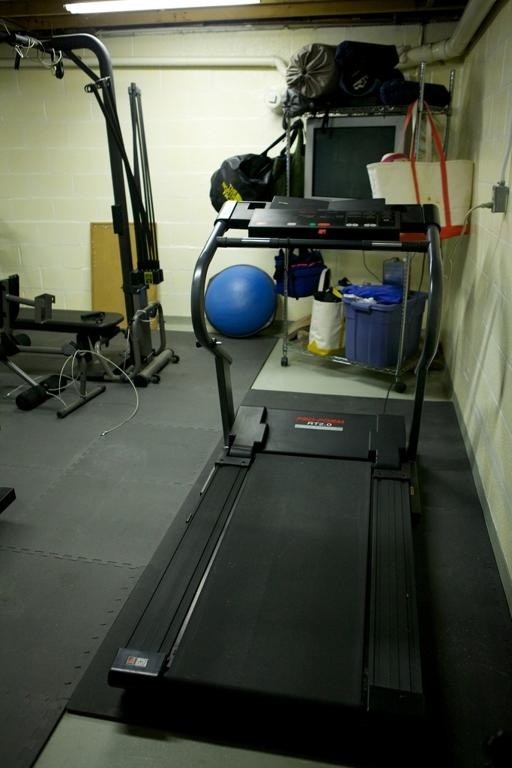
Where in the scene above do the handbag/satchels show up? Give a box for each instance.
[270,120,304,198]
[308,267,347,356]
[210,122,299,214]
[366,100,474,243]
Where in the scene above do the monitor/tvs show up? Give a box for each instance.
[304,116,404,211]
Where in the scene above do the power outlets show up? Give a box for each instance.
[492,184,508,214]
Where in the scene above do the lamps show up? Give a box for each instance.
[65,1,261,14]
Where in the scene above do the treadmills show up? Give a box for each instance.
[109,190,441,721]
